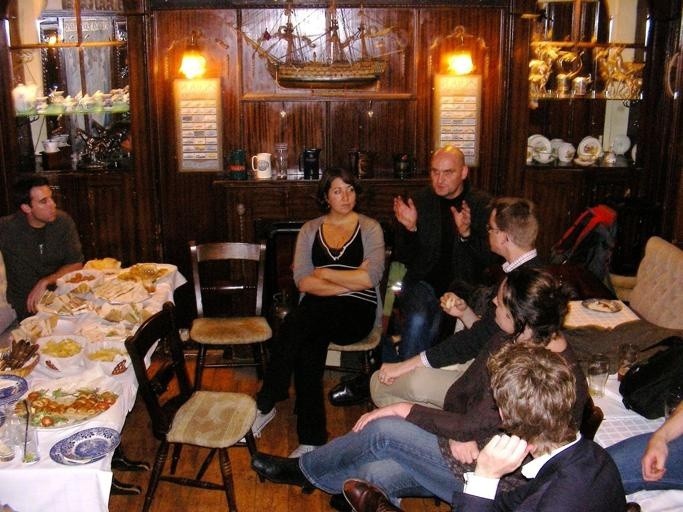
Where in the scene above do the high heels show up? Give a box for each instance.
[250,452,318,496]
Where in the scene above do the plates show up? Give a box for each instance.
[13,379,121,429]
[527,133,636,166]
[50,428,107,464]
[59,428,121,462]
[580,297,624,315]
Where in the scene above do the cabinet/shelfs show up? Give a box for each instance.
[519,1,654,170]
[4,172,143,270]
[521,163,653,279]
[213,174,445,361]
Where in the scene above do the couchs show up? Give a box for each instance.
[548,234,682,511]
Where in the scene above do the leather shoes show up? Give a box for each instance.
[328,374,374,407]
[342,478,402,511]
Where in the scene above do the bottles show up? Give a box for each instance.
[556,73,569,91]
[228,149,247,180]
[274,142,289,180]
[572,77,586,96]
[587,351,610,400]
[12,83,129,112]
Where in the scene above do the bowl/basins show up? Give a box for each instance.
[0,258,178,376]
[1,376,28,406]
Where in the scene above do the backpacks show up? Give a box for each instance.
[618,336,683,420]
[549,204,620,282]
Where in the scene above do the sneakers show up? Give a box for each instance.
[287,445,318,459]
[240,406,278,443]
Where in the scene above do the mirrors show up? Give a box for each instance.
[528,1,648,160]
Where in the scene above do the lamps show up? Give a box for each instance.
[443,23,479,78]
[172,26,210,78]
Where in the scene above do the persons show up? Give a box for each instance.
[0,177,85,324]
[368,196,545,413]
[236,165,386,458]
[342,339,627,512]
[393,145,497,362]
[603,399,683,492]
[250,264,593,512]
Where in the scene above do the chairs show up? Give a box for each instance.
[118,299,266,512]
[300,245,393,414]
[181,237,275,393]
[578,387,605,444]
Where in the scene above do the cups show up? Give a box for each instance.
[392,160,417,175]
[41,140,59,152]
[252,153,272,179]
[297,147,322,179]
[1,406,42,466]
[349,150,368,179]
[618,342,637,379]
[52,134,70,146]
